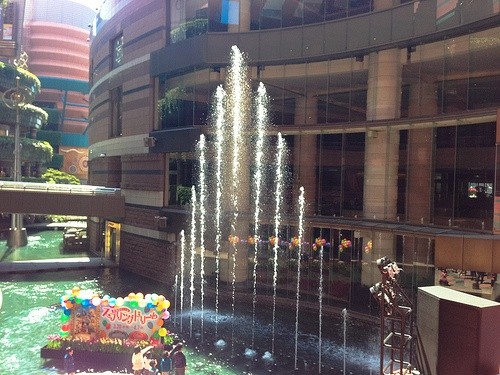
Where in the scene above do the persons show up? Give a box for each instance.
[63,347,76,375]
[131,344,154,375]
[144,341,173,375]
[480,186,486,219]
[437,267,498,286]
[171,342,187,375]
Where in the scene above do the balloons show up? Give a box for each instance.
[227,234,374,260]
[58,285,171,346]
